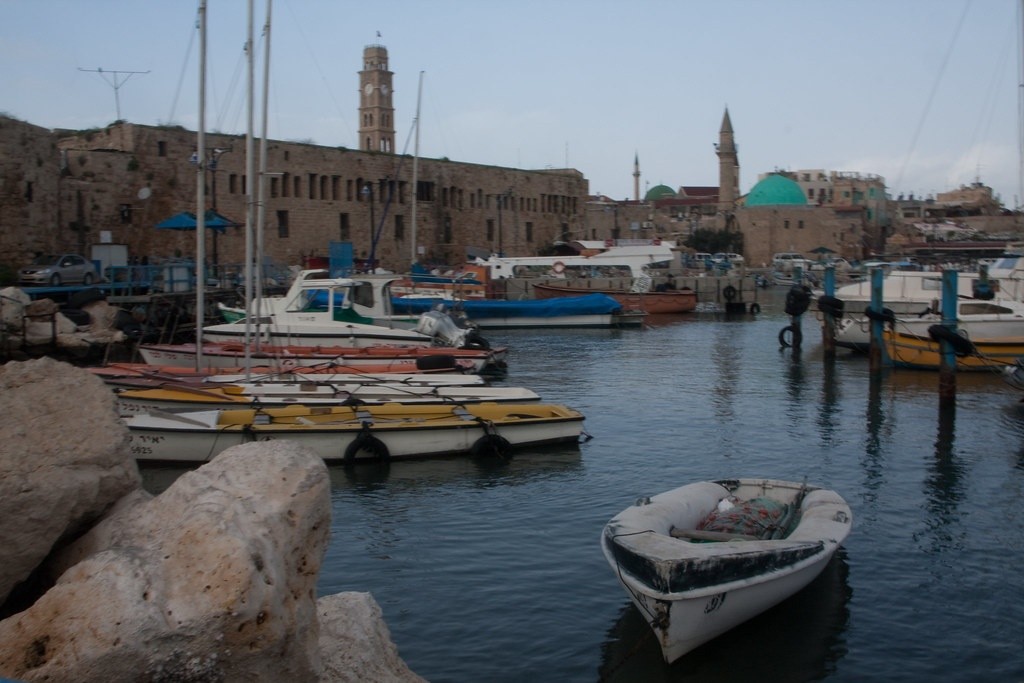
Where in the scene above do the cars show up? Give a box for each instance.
[17,252,98,286]
[692,252,745,264]
[772,253,807,269]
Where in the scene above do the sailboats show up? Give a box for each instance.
[89,0,509,388]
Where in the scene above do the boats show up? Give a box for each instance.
[832,300,1024,351]
[802,254,1024,315]
[304,290,648,328]
[531,281,697,312]
[880,325,1024,368]
[600,476,853,664]
[118,389,543,415]
[127,402,587,463]
